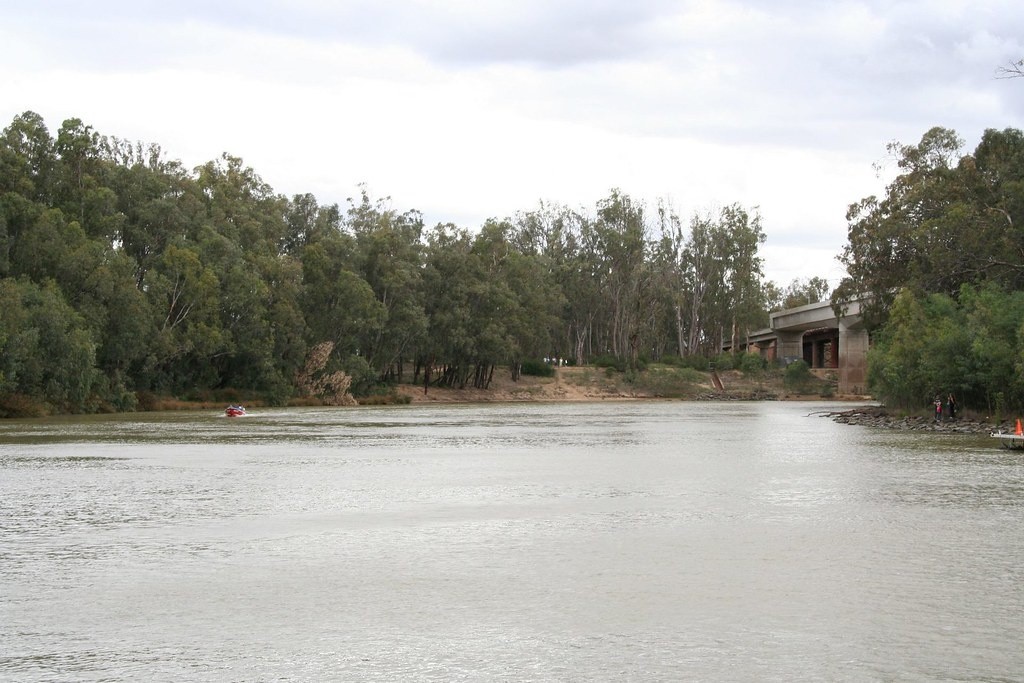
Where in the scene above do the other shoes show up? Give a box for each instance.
[950,416,953,420]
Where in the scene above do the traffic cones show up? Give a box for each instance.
[1015,419,1022,435]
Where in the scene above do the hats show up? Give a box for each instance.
[938,402,942,405]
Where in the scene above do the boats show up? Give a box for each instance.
[225,406,246,418]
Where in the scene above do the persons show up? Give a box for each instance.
[947,393,956,419]
[932,395,942,422]
[544,356,568,368]
